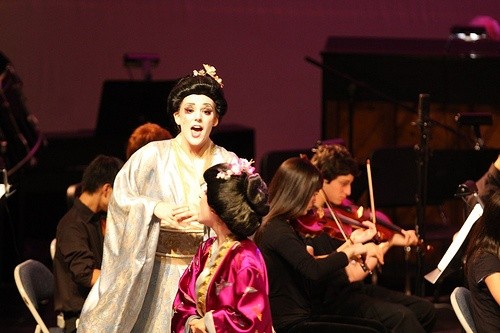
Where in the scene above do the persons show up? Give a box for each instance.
[464,188,500,333]
[126,122,173,160]
[78,63,241,333]
[292,143,419,259]
[171,158,273,333]
[247,158,438,333]
[22,144,72,244]
[52,154,125,333]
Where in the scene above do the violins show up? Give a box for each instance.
[294,196,438,262]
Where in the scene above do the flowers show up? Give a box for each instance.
[216,157,256,179]
[193,64,224,88]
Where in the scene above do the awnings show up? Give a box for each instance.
[320,35,500,104]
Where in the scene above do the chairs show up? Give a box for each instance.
[450,287,477,333]
[14,259,66,333]
